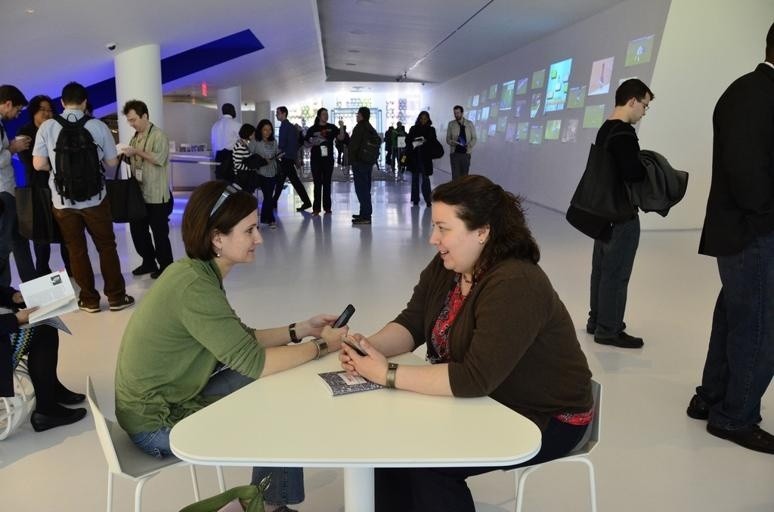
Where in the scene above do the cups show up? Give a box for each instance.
[14,135,25,143]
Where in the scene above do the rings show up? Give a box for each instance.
[342,333,347,337]
[348,348,355,353]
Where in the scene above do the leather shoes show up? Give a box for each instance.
[31,406,86,431]
[297,204,332,216]
[594,332,643,348]
[57,394,85,404]
[707,423,774,453]
[587,321,626,333]
[686,395,762,422]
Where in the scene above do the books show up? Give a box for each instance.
[18,269,79,325]
[319,368,386,398]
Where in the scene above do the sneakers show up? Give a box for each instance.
[352,215,371,224]
[413,201,431,207]
[151,269,163,278]
[269,223,276,229]
[133,261,158,275]
[78,300,100,312]
[109,294,134,310]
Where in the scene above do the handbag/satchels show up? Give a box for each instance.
[179,485,265,511]
[108,176,146,223]
[423,138,444,159]
[14,186,61,242]
[566,143,633,238]
[359,134,379,165]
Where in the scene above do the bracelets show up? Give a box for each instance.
[312,338,328,360]
[290,323,302,342]
[385,362,398,390]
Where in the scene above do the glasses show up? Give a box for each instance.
[209,183,242,216]
[638,100,649,111]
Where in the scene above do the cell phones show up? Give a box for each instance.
[332,304,356,328]
[344,336,367,356]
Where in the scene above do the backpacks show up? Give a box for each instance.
[50,115,102,201]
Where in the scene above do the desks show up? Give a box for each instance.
[165,350,544,509]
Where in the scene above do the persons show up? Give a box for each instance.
[17,94,74,276]
[586,78,655,348]
[118,99,175,279]
[0,84,41,293]
[210,102,477,229]
[115,178,348,512]
[338,174,594,512]
[0,285,88,431]
[32,82,135,312]
[688,22,773,456]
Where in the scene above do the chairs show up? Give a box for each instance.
[86,371,230,512]
[503,376,604,512]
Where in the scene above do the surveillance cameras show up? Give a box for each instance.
[245,103,247,105]
[107,45,116,50]
[396,75,403,82]
